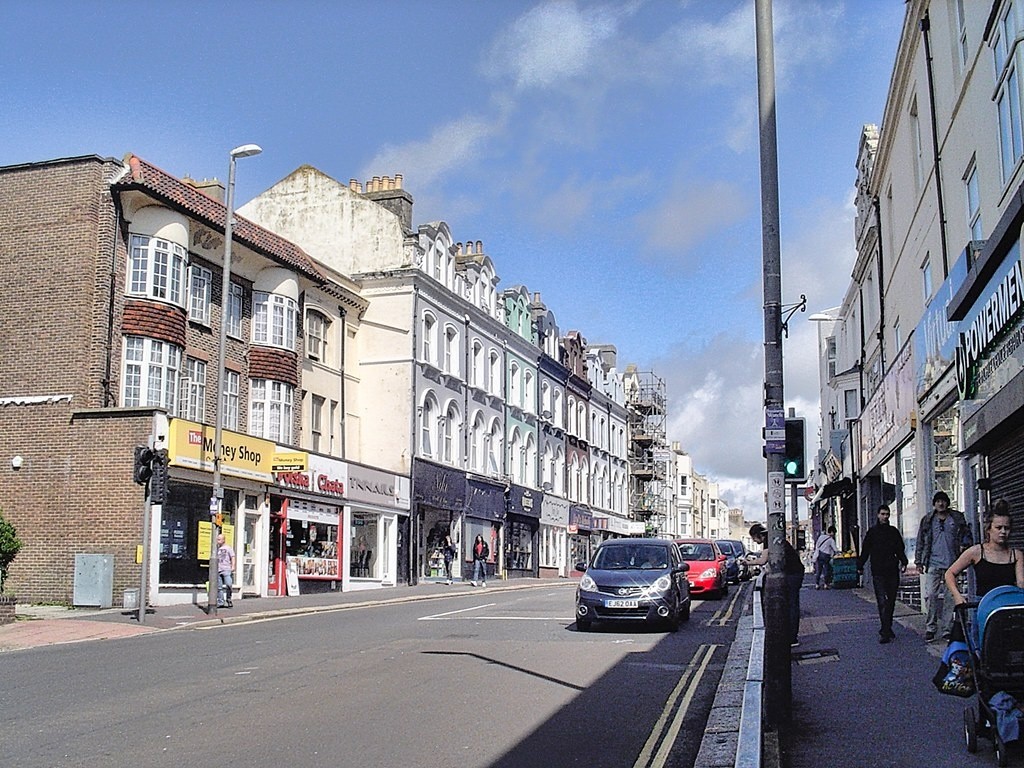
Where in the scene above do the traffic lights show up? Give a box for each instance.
[784,416,809,484]
[133,445,154,483]
[150,447,173,507]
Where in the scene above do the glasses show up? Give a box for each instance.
[939,519,944,531]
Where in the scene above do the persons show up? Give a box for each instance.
[737,524,806,647]
[856,505,908,645]
[913,491,973,643]
[470,534,490,588]
[815,526,839,589]
[944,499,1024,608]
[443,536,456,585]
[217,534,235,608]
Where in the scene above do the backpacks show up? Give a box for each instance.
[932,609,978,698]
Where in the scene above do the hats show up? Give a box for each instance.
[748,523,769,535]
[933,492,951,506]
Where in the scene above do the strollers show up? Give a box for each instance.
[950,584,1024,768]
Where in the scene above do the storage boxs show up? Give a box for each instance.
[832,557,859,589]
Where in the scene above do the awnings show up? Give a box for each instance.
[811,477,851,504]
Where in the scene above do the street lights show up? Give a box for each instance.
[205,144,263,616]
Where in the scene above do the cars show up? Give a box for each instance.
[715,541,742,585]
[719,539,746,558]
[574,539,692,631]
[676,539,729,601]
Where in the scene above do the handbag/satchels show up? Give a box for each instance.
[812,548,820,563]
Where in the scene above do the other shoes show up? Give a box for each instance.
[481,582,486,587]
[824,585,832,589]
[943,635,950,641]
[791,639,800,647]
[879,629,895,644]
[217,605,226,608]
[925,632,934,642]
[816,584,821,590]
[226,599,233,607]
[471,581,477,587]
